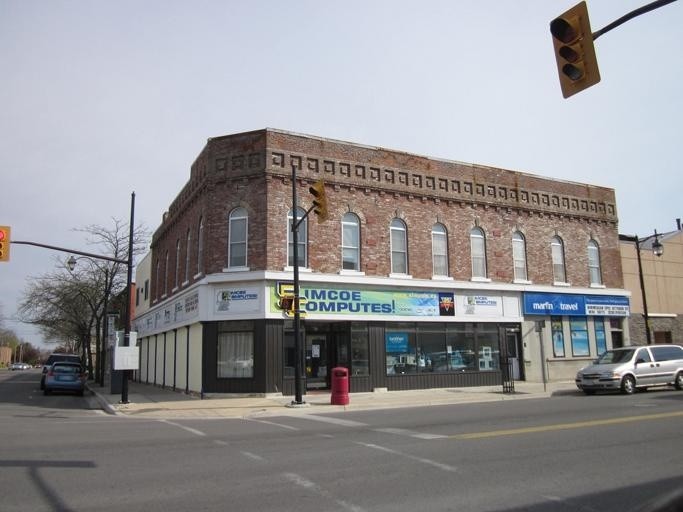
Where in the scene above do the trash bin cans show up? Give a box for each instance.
[331,367,349,405]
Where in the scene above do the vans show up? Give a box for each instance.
[575,343,683,395]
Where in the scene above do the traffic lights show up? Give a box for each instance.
[310,177,329,225]
[0,225,11,262]
[280,295,293,310]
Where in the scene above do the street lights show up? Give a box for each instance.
[67,254,109,388]
[634,228,665,345]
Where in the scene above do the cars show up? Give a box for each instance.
[11,351,86,396]
